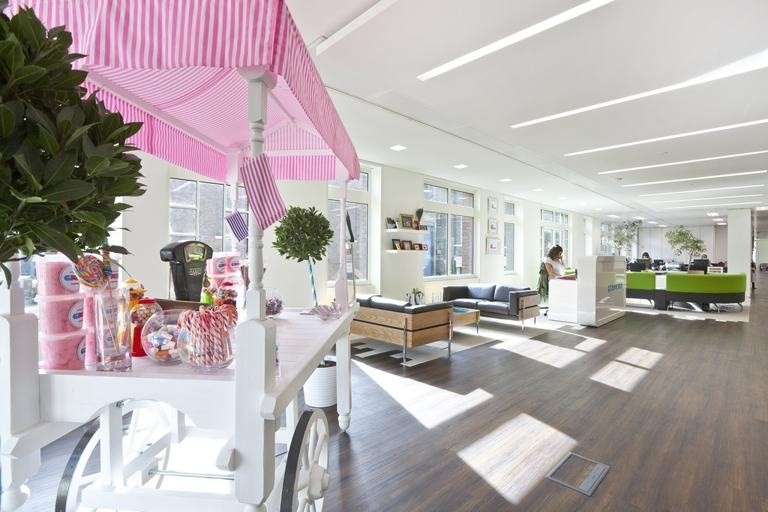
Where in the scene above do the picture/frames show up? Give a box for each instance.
[392,238,428,251]
[399,213,416,229]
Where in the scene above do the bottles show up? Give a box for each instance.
[84,290,238,371]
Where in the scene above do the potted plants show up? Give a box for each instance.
[272,205,336,409]
[410,287,422,304]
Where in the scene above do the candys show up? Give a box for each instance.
[131,303,238,369]
[72,250,119,352]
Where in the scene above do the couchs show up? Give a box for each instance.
[442,283,541,331]
[350,295,454,365]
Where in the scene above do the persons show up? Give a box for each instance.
[701,254,707,260]
[642,252,652,263]
[545,245,567,280]
[751,261,757,289]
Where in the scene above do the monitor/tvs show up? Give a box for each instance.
[630,264,646,272]
[654,260,663,270]
[694,259,709,274]
[637,259,651,270]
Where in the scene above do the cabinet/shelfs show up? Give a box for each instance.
[385,229,431,254]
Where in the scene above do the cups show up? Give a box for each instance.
[266,288,287,316]
[432,292,439,303]
